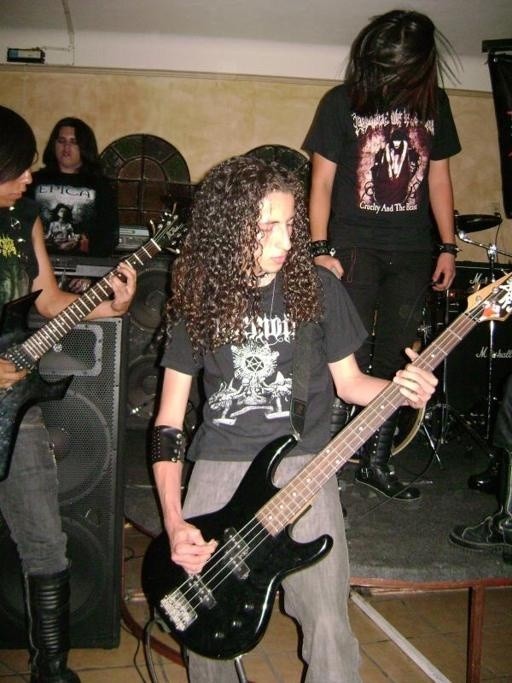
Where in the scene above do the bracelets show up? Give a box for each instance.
[440,241,460,256]
[310,240,331,256]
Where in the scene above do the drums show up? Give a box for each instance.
[344,330,427,463]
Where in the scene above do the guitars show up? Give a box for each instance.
[0,203,190,480]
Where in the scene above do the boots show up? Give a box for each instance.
[330,398,422,502]
[467,448,508,492]
[19,559,82,682]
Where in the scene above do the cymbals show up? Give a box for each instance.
[453,213,502,234]
[141,271,512,658]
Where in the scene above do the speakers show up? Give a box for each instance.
[125,248,205,539]
[0,315,132,650]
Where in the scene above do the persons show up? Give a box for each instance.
[0,105,140,682]
[450,374,512,565]
[303,9,461,502]
[152,157,439,683]
[31,115,121,294]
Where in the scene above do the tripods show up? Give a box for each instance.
[422,290,496,467]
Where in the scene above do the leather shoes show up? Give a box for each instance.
[448,503,512,562]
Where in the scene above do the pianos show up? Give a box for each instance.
[48,252,125,277]
